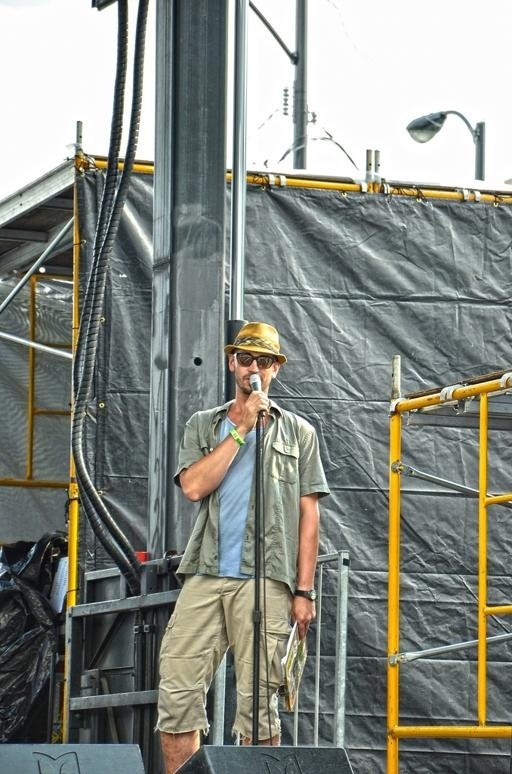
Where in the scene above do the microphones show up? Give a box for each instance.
[250,374,265,416]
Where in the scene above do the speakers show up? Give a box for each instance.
[173,745,355,774]
[0,744,146,774]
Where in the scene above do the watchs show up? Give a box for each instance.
[294,590,317,601]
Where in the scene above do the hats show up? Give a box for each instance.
[223,322,287,365]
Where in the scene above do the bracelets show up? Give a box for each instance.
[230,428,246,446]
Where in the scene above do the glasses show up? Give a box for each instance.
[236,352,276,369]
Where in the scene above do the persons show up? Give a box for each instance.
[154,322,332,774]
